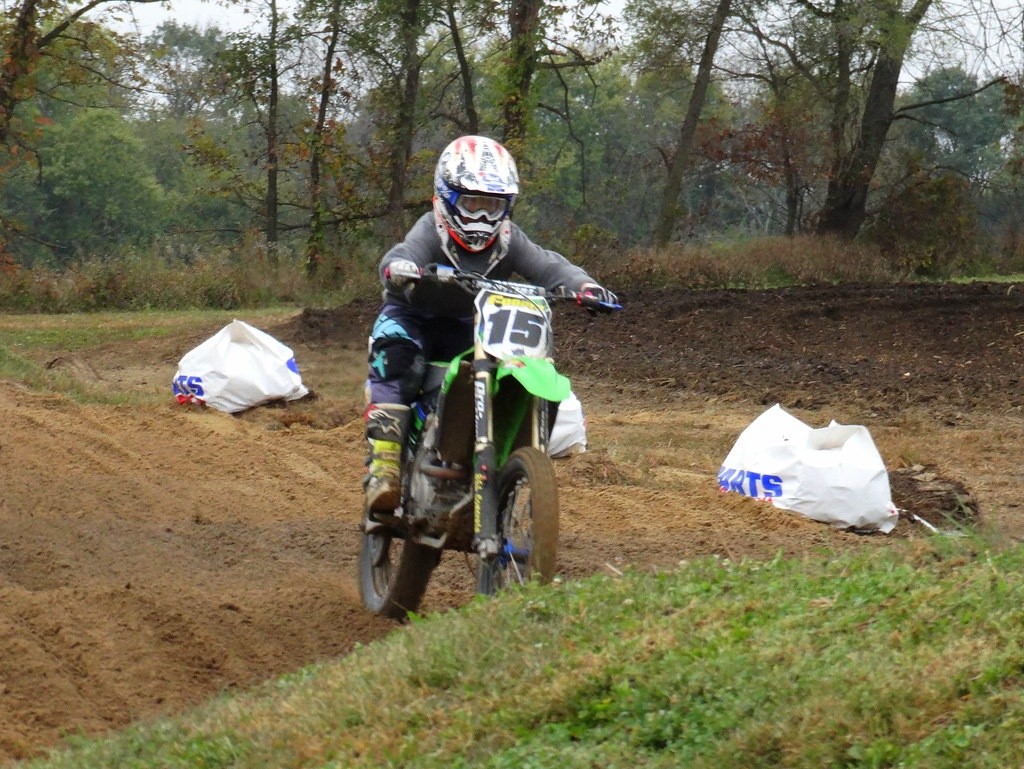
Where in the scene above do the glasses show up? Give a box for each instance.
[453,193,508,223]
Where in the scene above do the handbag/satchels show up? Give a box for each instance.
[173,320,309,415]
[716,405,898,535]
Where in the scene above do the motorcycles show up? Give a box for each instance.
[359,262,624,622]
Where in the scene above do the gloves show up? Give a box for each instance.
[389,260,419,287]
[580,283,618,318]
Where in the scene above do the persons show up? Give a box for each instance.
[364,135,619,525]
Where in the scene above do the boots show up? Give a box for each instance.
[362,403,410,513]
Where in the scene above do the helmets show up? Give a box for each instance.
[432,134,519,252]
